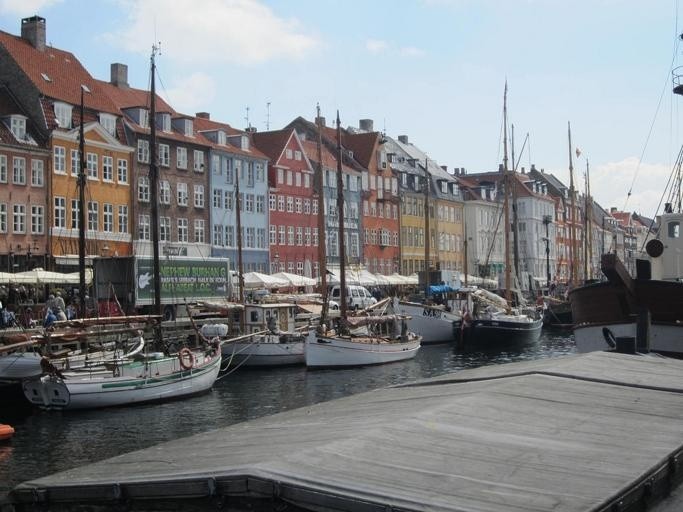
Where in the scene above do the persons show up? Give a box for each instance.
[527,275,570,319]
[460,304,473,333]
[422,294,451,317]
[204,335,222,358]
[267,317,277,335]
[0,280,124,326]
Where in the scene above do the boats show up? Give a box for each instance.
[1,347,683,511]
[567,12,683,360]
[0,423,16,439]
